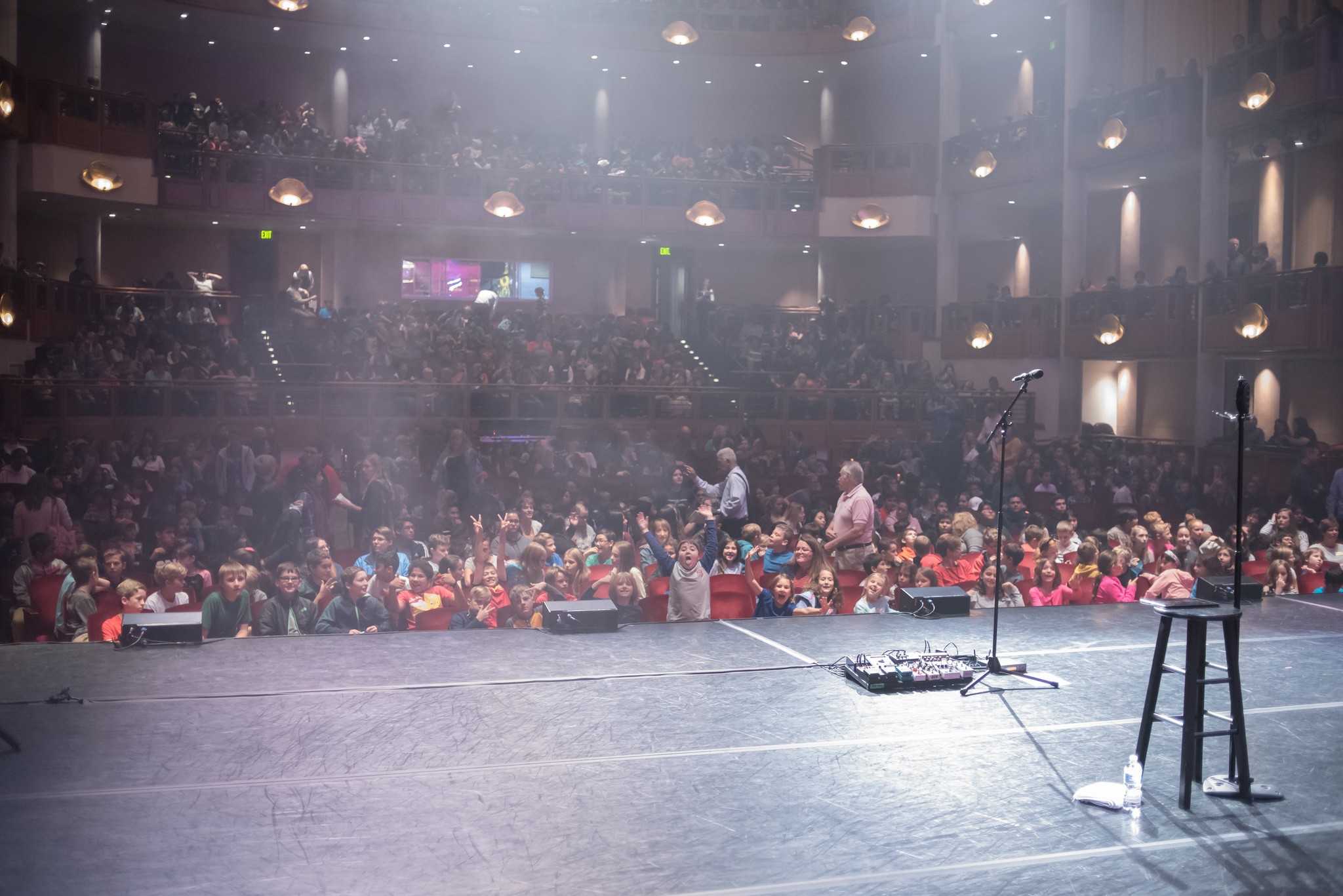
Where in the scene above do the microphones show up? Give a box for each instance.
[1012,369,1044,383]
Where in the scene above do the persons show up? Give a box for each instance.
[0,93,1343,643]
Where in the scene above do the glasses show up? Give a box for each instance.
[1282,538,1296,542]
[372,537,386,542]
[988,544,1004,550]
[278,575,299,582]
[594,539,608,545]
[320,543,330,551]
[982,508,997,513]
[793,548,814,554]
[402,525,416,530]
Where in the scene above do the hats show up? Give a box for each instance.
[968,497,983,510]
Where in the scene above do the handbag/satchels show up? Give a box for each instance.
[46,498,79,560]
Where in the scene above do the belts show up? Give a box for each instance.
[837,541,872,551]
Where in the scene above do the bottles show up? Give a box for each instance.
[1123,755,1143,812]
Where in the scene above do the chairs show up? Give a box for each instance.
[36,544,1326,643]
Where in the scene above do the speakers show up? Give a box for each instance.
[1196,575,1264,603]
[898,586,971,617]
[541,599,619,631]
[121,611,203,646]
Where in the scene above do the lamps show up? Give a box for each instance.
[0,0,1276,350]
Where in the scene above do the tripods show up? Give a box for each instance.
[960,379,1059,696]
